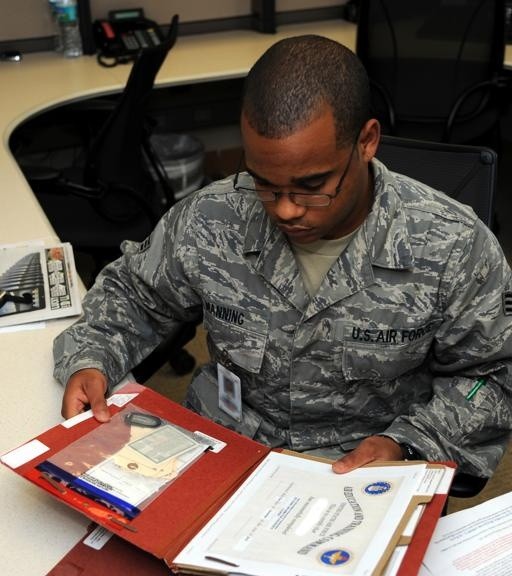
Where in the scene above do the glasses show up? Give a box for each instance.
[233,134,359,206]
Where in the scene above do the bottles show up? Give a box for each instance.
[48,0,83,57]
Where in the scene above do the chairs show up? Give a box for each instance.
[374,135,497,518]
[356,0,512,237]
[25,15,196,377]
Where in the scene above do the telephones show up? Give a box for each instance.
[92,8,167,59]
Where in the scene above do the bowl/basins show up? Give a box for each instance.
[148,133,205,200]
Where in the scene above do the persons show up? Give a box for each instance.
[53,34,512,518]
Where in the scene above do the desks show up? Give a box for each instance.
[0,19,512,576]
